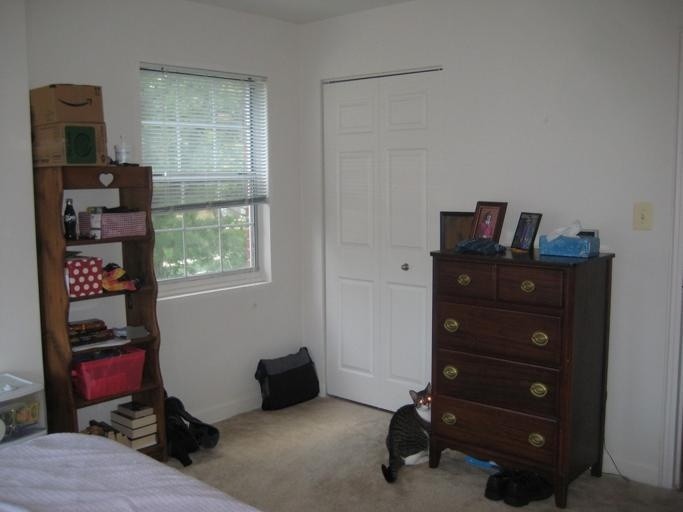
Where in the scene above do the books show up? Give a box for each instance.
[110,401,158,450]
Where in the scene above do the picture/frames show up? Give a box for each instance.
[439,211,479,249]
[509,212,541,252]
[469,199,508,246]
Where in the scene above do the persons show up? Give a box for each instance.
[476,211,494,238]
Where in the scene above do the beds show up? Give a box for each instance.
[2,424,262,510]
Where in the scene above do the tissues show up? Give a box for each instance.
[539,211,600,258]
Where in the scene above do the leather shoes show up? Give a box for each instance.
[503,472,556,507]
[485,469,518,501]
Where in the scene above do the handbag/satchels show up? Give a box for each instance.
[254,345,320,412]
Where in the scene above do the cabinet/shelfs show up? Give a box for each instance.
[428,244,614,511]
[34,163,168,461]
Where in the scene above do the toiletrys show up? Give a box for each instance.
[114,135,132,164]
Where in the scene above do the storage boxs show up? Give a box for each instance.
[0,370,48,452]
[65,252,104,299]
[29,83,105,128]
[30,121,107,168]
[72,346,146,403]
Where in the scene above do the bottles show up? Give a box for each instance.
[112,133,132,163]
[63,199,77,239]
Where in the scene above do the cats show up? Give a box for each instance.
[381,382,432,484]
